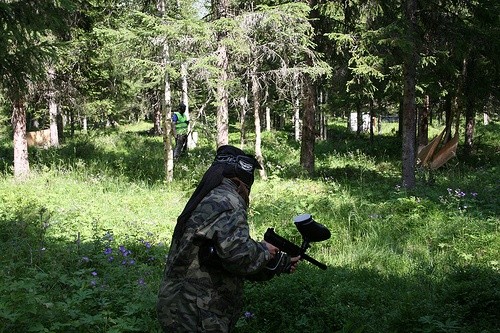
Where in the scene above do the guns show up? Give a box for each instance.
[262,214,331,274]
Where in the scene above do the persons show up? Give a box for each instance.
[156,145,300,333]
[171,103,189,162]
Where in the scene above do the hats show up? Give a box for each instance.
[213,145,262,185]
